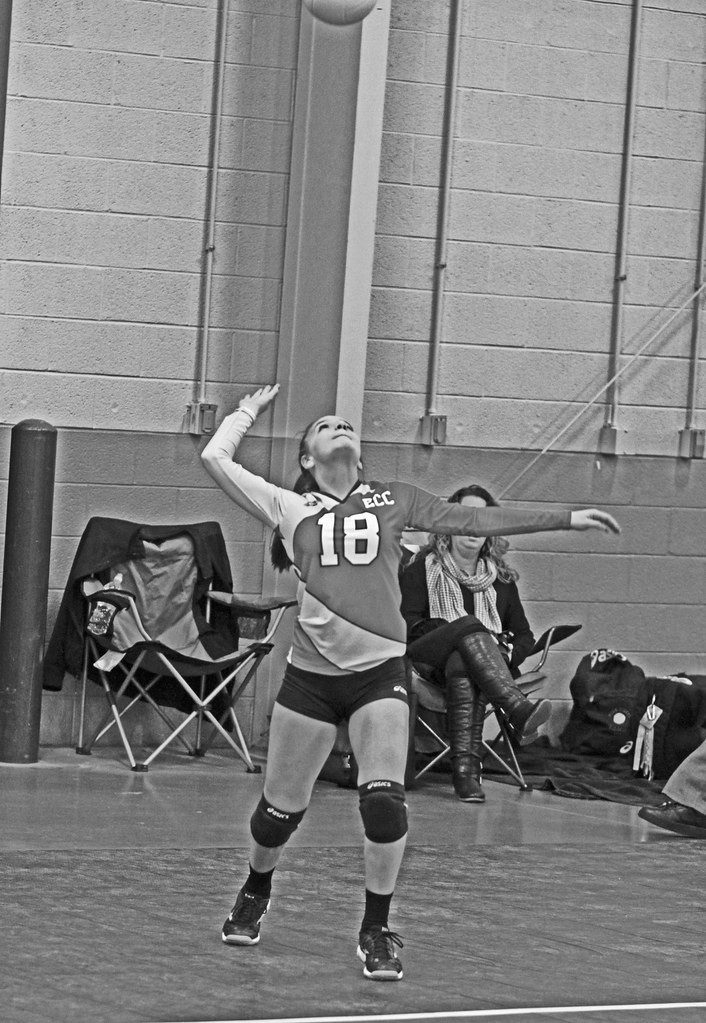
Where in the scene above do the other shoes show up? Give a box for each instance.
[637,803,706,837]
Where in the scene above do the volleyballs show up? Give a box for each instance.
[304,0,376,26]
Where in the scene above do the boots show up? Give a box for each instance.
[460,631,551,747]
[446,675,488,803]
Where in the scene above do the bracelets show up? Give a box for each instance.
[235,406,256,427]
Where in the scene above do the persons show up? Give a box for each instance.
[639,740,706,841]
[198,383,621,978]
[398,483,554,804]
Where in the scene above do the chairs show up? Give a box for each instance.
[73,516,301,778]
[398,542,582,794]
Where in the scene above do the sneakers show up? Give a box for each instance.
[222,888,272,944]
[354,923,405,981]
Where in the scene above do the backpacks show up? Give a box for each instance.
[561,648,644,756]
